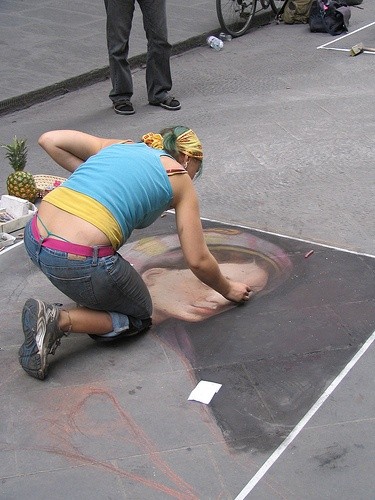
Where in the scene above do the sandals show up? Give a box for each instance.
[114,101,135,115]
[149,94,181,110]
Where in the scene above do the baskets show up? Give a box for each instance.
[33,174,67,197]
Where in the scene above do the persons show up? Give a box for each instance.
[19,125,254,380]
[104,0,181,115]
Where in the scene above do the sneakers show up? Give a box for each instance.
[18,298,64,380]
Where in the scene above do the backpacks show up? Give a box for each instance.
[309,0,351,36]
[283,0,315,24]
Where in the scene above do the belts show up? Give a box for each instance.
[31,211,115,257]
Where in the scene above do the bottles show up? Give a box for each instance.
[206,36,224,51]
[219,33,231,41]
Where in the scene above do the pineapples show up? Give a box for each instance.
[0,135,37,204]
